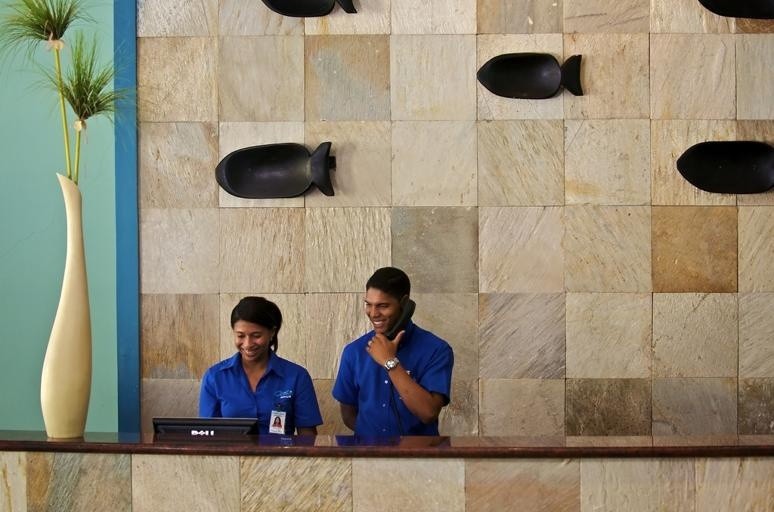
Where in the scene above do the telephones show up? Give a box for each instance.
[388,297,416,343]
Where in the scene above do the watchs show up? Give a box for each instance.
[383,356,400,371]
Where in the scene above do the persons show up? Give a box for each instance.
[198,297,323,434]
[271,416,284,428]
[332,268,454,435]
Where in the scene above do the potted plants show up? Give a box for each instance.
[0,0,164,441]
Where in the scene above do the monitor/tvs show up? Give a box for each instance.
[150,414,259,435]
